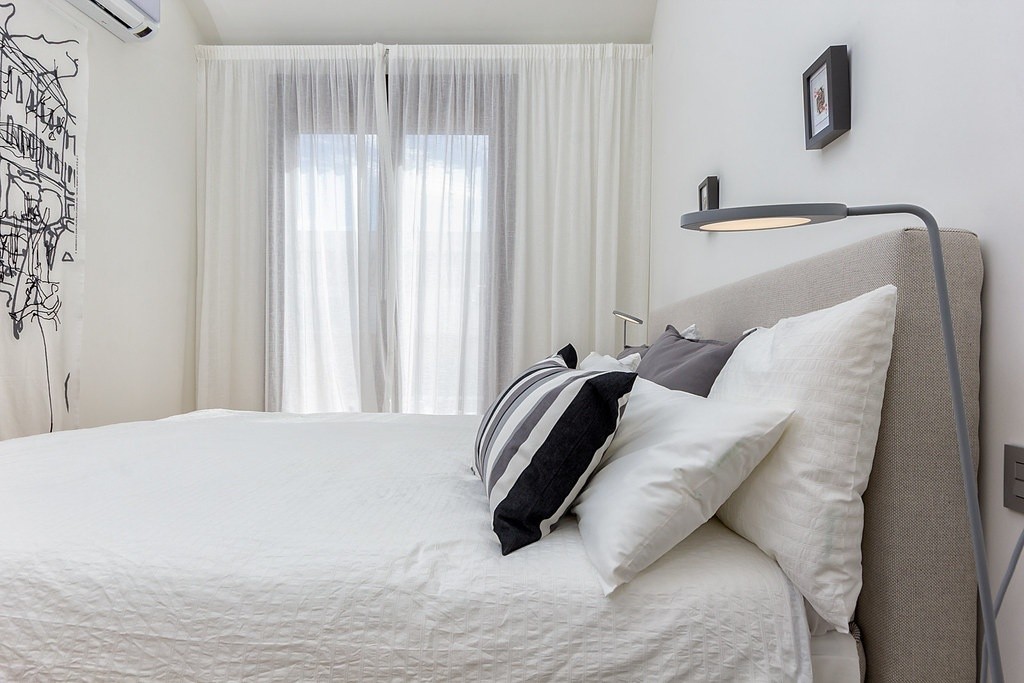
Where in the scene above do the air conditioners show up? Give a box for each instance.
[66,0,161,46]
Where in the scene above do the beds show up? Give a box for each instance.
[0,224,987,683]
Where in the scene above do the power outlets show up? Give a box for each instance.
[1003,444,1024,513]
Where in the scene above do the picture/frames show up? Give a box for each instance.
[697,176,719,211]
[802,44,852,150]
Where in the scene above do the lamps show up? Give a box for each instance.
[612,310,643,350]
[680,203,1005,683]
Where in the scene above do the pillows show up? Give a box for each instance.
[636,324,757,396]
[617,344,649,358]
[618,353,641,371]
[469,342,638,556]
[707,284,897,635]
[578,352,625,372]
[574,369,795,598]
